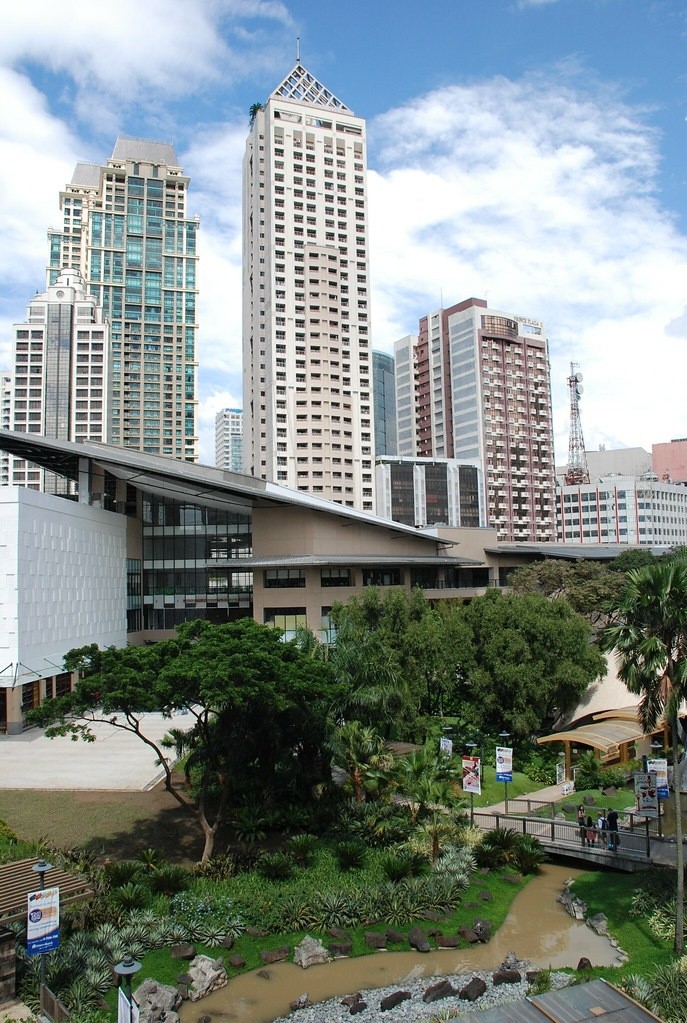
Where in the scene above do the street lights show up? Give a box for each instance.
[498,730,511,816]
[32,858,53,983]
[639,754,652,858]
[114,956,143,1023]
[466,741,478,829]
[650,740,664,837]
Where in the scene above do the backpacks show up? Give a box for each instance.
[599,817,608,830]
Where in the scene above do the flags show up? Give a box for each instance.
[633,771,660,819]
[646,758,670,799]
[27,887,59,957]
[461,756,481,796]
[496,747,513,782]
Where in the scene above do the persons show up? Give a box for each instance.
[578,805,620,851]
